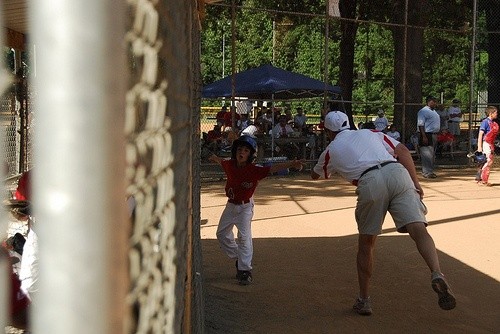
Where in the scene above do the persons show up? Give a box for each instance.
[15,172,32,204]
[416,95,441,178]
[437,99,462,147]
[203,135,307,285]
[310,111,455,316]
[208,101,313,160]
[358,109,401,141]
[19,225,39,303]
[473,105,499,186]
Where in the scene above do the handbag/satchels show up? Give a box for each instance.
[466,150,487,169]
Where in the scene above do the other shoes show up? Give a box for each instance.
[424,173,438,179]
[474,180,491,187]
[357,160,396,180]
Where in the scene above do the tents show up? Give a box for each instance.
[201,62,346,161]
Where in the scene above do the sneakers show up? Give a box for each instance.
[353,296,373,314]
[431,271,456,311]
[239,271,254,286]
[236,258,239,278]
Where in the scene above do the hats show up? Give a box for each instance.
[319,111,350,131]
[426,95,436,102]
[378,109,384,114]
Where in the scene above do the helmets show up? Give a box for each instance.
[232,135,256,163]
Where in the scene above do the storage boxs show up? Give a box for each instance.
[262,156,289,175]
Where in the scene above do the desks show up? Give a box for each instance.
[255,134,309,159]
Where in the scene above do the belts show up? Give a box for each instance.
[417,132,438,134]
[228,199,249,204]
